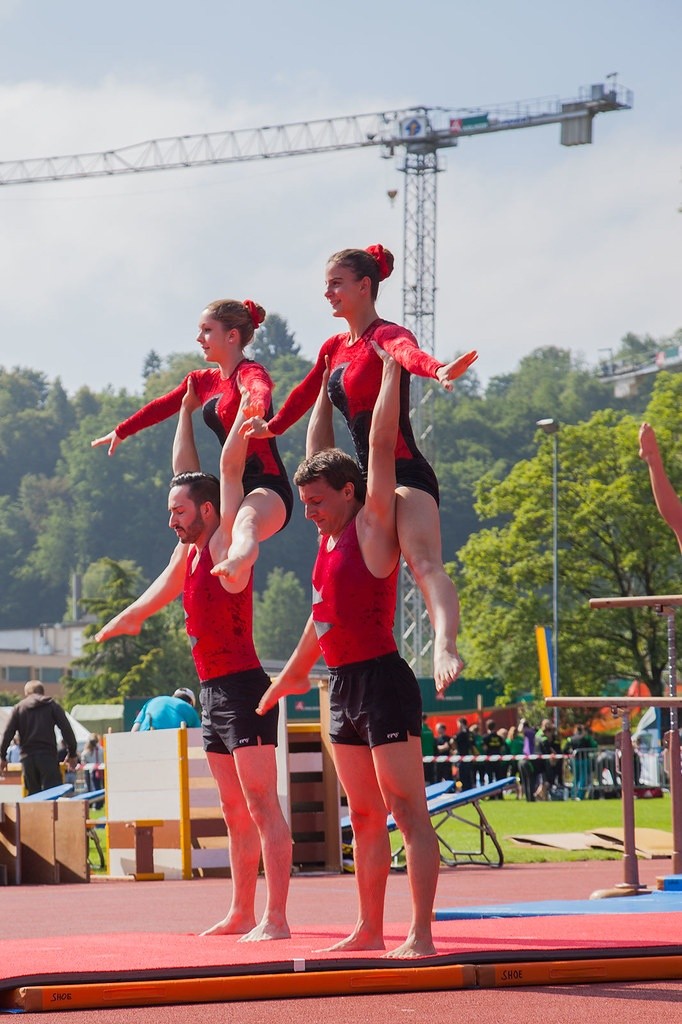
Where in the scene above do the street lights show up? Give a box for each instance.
[537,418,560,729]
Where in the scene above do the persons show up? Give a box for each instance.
[310,338,440,959]
[168,377,294,942]
[238,245,480,714]
[5,731,104,811]
[131,687,201,732]
[91,300,293,642]
[638,421,682,550]
[0,680,77,796]
[421,711,641,801]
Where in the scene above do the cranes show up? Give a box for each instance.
[0,72,632,677]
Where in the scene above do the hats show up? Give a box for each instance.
[175,687,196,705]
